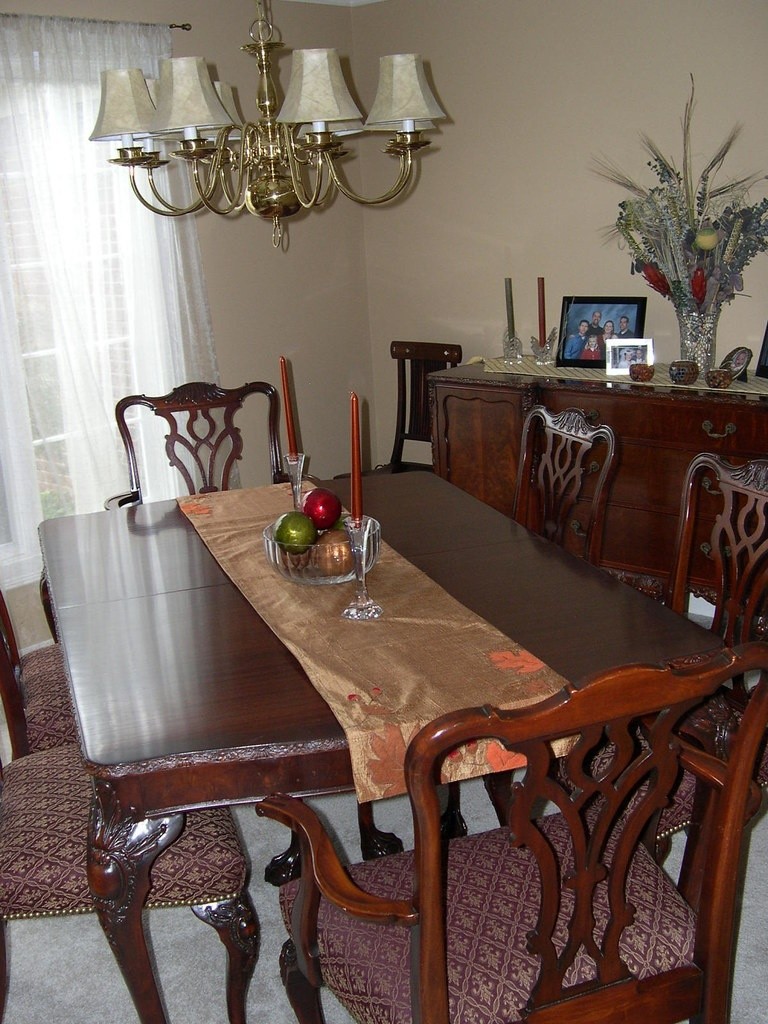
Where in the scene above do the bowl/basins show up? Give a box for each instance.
[706,368,731,388]
[669,359,698,385]
[263,512,381,585]
[630,363,654,382]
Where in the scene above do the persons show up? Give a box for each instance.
[564,311,633,360]
[731,351,746,372]
[619,348,646,368]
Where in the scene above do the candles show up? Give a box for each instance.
[280,354,299,455]
[351,390,363,519]
[537,276,547,345]
[506,278,516,340]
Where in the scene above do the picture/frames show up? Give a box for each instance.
[606,337,654,374]
[555,296,648,367]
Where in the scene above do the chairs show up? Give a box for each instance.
[103,381,320,511]
[482,451,768,867]
[251,641,768,1024]
[0,743,262,1024]
[334,340,462,479]
[0,589,78,759]
[507,403,620,568]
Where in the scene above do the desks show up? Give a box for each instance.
[37,472,724,1022]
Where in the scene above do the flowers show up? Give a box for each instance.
[587,69,768,314]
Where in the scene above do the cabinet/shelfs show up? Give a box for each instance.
[429,367,768,639]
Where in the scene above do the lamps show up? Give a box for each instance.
[89,0,447,247]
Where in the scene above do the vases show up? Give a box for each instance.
[677,309,721,378]
